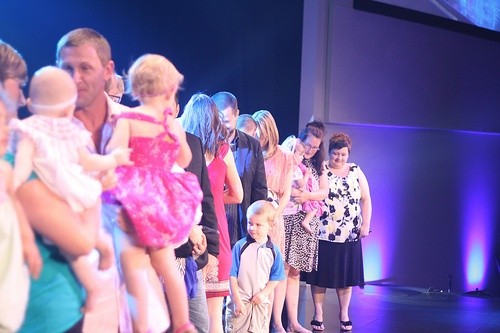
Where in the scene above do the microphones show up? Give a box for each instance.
[349,229,372,240]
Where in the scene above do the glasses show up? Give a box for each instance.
[305,144,317,150]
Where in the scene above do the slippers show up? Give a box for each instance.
[340,320,352,331]
[311,321,324,331]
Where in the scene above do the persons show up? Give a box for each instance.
[209,92,268,251]
[235,114,255,137]
[300,132,373,331]
[306,121,324,135]
[105,55,202,333]
[167,94,244,333]
[13,66,134,271]
[272,125,329,333]
[252,108,293,333]
[159,162,208,299]
[0,91,42,333]
[280,136,318,235]
[106,73,124,105]
[0,40,101,333]
[57,28,130,156]
[224,200,287,333]
